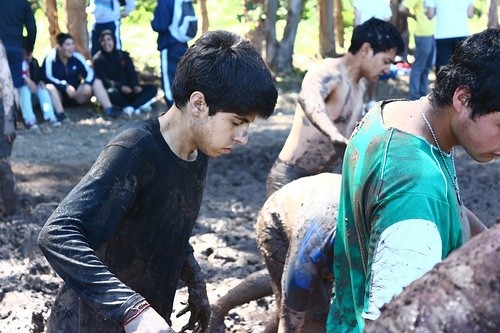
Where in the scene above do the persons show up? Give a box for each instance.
[149,0,199,112]
[35,28,280,333]
[0,34,31,222]
[40,32,125,124]
[200,14,409,333]
[359,0,437,102]
[0,0,37,119]
[16,36,64,132]
[363,216,500,333]
[323,22,500,333]
[90,28,166,121]
[424,0,476,74]
[253,170,345,333]
[83,0,136,69]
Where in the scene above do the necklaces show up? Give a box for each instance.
[416,92,466,207]
